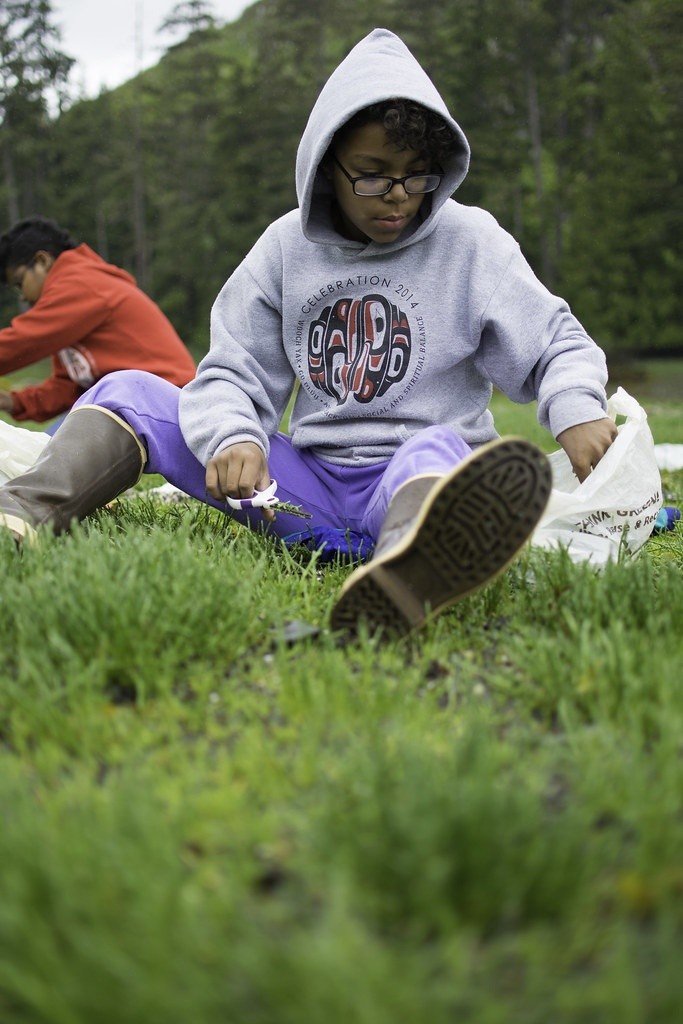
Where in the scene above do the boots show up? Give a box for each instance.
[329,436,553,652]
[0,405,148,552]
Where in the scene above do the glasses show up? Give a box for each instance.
[6,260,36,296]
[327,148,445,196]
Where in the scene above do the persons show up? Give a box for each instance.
[0,25,617,655]
[0,218,196,487]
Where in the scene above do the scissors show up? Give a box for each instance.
[225,478,313,520]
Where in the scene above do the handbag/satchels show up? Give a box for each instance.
[527,386,663,571]
